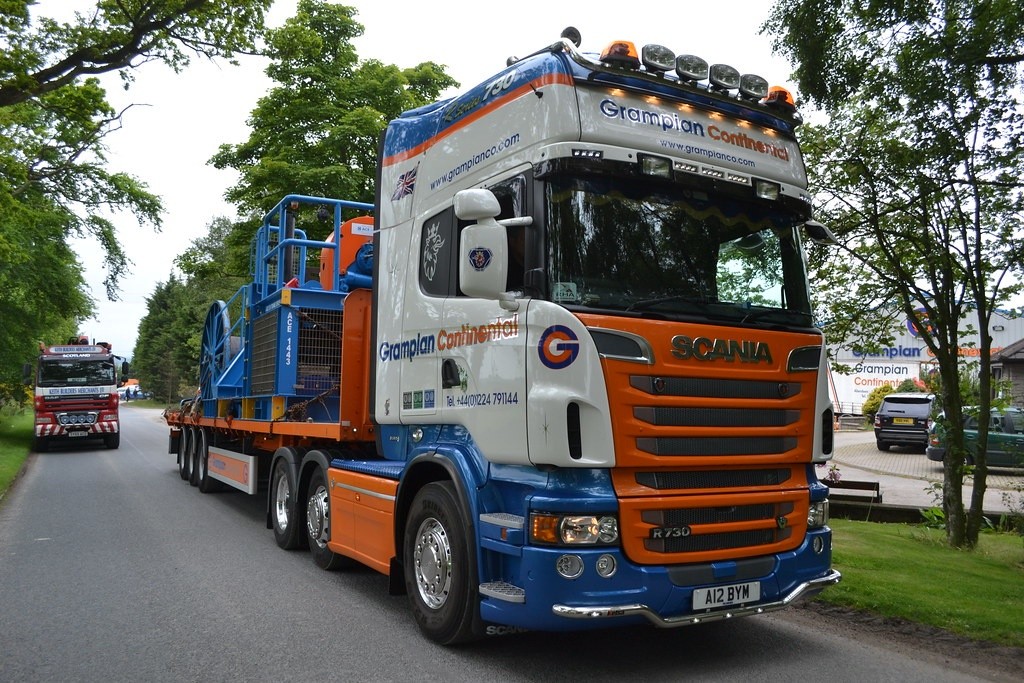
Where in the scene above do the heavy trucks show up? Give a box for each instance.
[22,337,130,449]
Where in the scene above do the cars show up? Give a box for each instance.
[117,385,132,398]
[120,389,147,401]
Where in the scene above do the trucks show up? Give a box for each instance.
[165,25,842,649]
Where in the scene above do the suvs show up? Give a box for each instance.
[874,391,937,451]
[925,406,1024,467]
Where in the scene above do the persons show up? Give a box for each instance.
[126,387,130,401]
[133,389,140,402]
